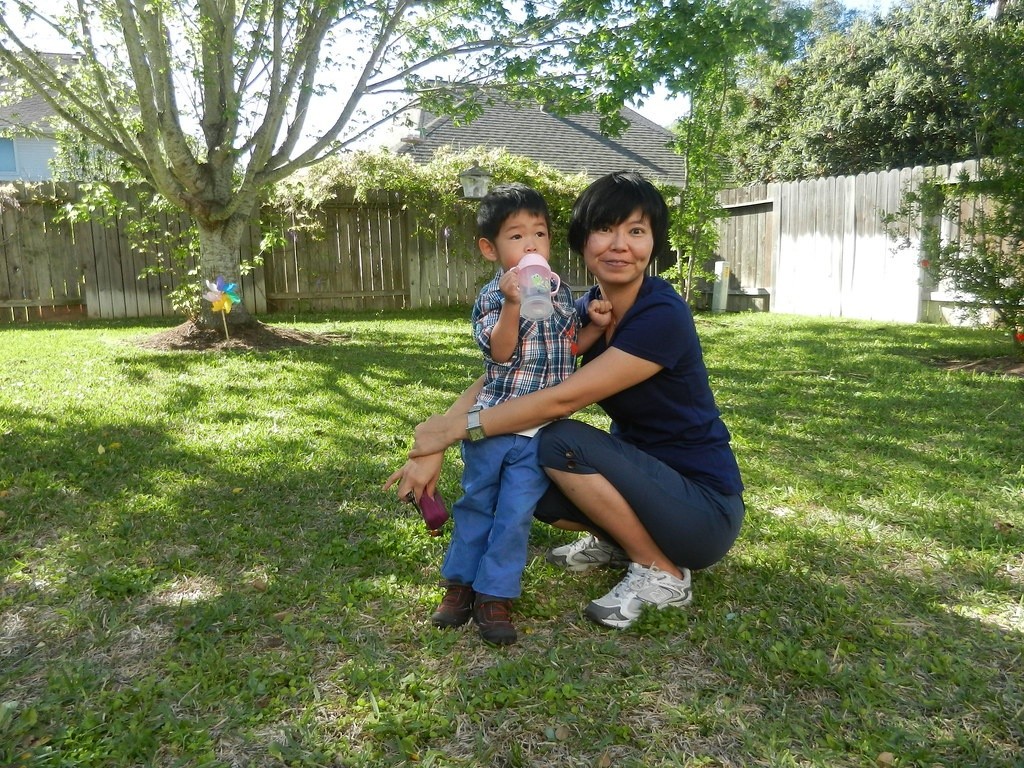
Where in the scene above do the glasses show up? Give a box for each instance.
[397,477,421,516]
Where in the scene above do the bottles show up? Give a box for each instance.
[508,254,560,323]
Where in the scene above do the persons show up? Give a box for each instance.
[430,183,613,646]
[382,169,746,628]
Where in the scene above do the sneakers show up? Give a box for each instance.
[585,561,693,631]
[548,534,631,572]
[431,579,474,630]
[473,592,517,648]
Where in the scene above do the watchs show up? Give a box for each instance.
[466,405,488,444]
